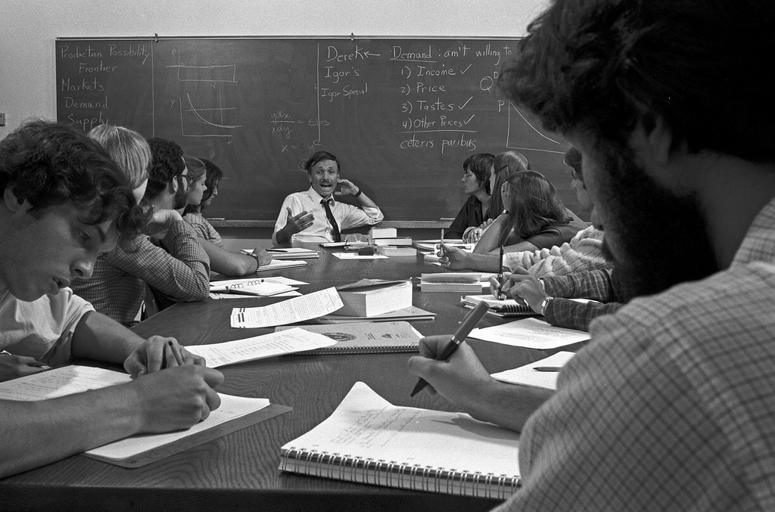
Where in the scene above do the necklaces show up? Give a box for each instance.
[178,155,206,215]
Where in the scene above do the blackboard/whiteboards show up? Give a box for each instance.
[54,34,593,228]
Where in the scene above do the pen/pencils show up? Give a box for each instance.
[411,300,491,398]
[498,244,504,298]
[265,249,289,252]
[533,367,562,372]
[440,228,444,256]
[168,340,184,366]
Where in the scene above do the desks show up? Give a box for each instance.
[1,245,589,510]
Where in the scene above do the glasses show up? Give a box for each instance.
[462,174,475,181]
[180,174,190,180]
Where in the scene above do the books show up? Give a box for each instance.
[413,237,477,255]
[330,278,413,318]
[420,271,483,294]
[369,227,419,259]
[277,380,523,501]
[273,320,426,356]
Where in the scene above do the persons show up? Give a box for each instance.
[141,137,273,277]
[405,2,775,511]
[1,121,225,479]
[183,158,223,248]
[438,146,671,333]
[272,152,385,245]
[70,122,212,326]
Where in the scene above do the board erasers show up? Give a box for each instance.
[206,217,226,221]
[440,217,456,221]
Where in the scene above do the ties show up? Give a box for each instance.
[322,201,341,241]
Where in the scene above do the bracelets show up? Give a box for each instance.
[352,187,361,197]
[247,253,259,272]
